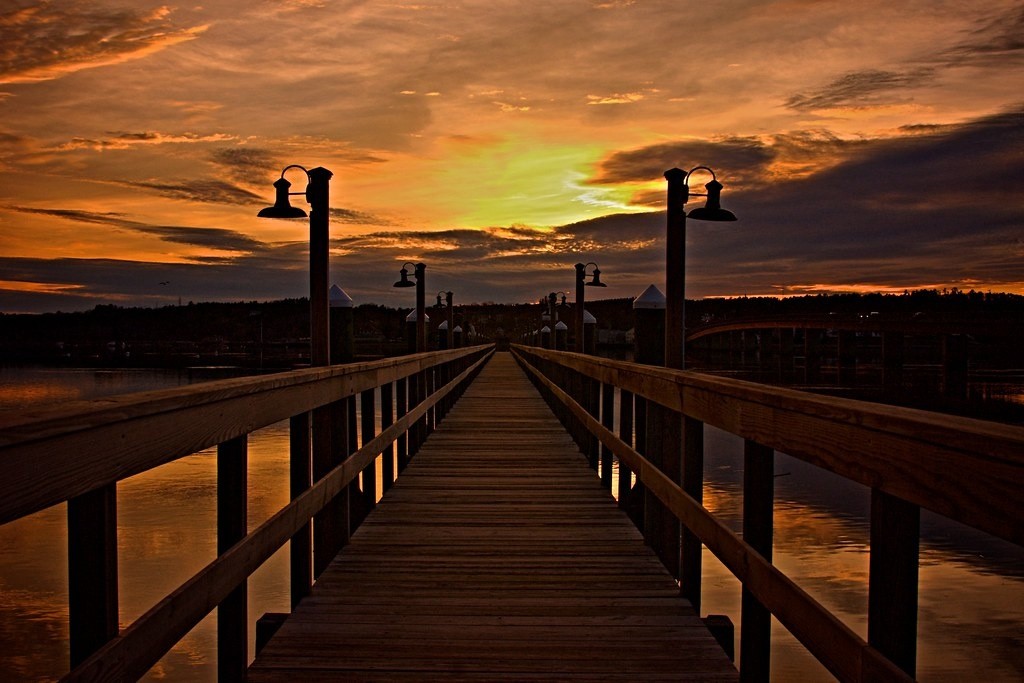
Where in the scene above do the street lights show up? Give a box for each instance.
[259,163,335,579]
[573,262,608,453]
[393,262,426,455]
[663,165,739,589]
[433,291,453,349]
[516,291,571,349]
